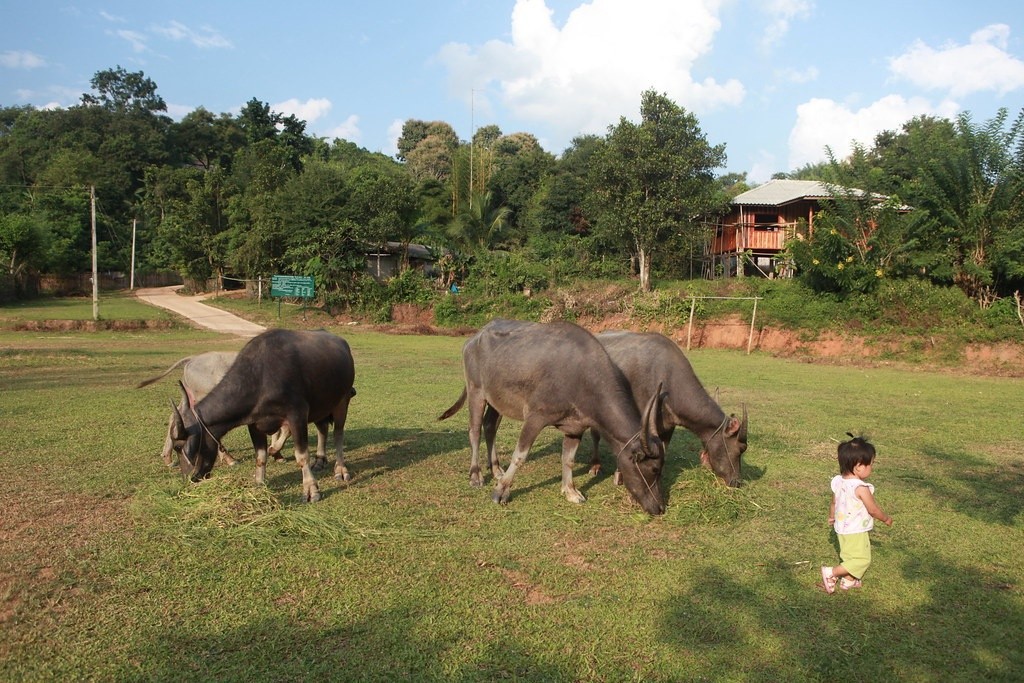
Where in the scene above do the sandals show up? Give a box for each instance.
[821,566,839,594]
[840,577,862,590]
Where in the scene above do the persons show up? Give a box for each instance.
[821,432,892,594]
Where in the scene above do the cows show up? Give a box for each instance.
[438,318,750,517]
[134,326,358,503]
